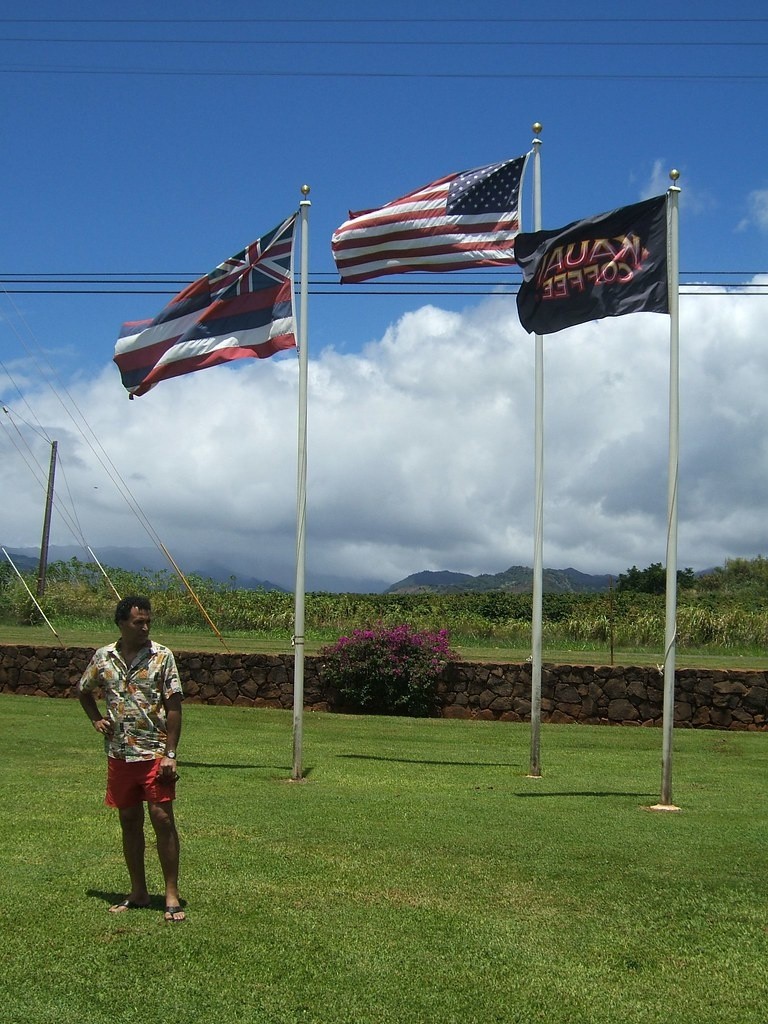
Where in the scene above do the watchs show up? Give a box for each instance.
[163,750,176,759]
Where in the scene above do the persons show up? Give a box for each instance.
[77,596,184,921]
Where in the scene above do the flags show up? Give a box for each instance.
[332,156,532,286]
[112,214,300,400]
[513,193,672,336]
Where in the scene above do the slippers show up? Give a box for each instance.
[164,905,186,922]
[108,899,153,913]
